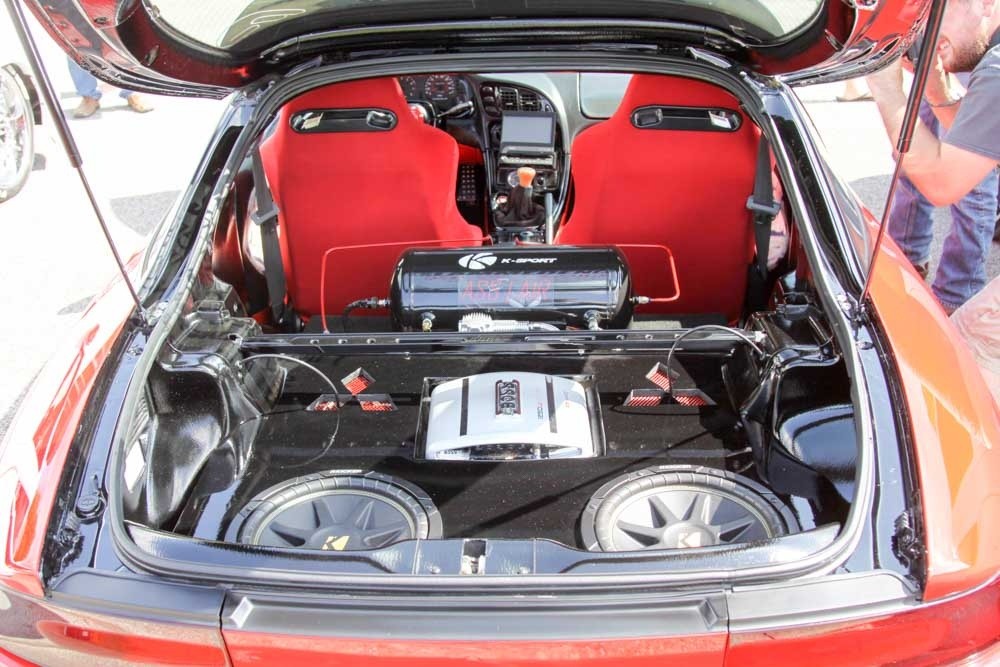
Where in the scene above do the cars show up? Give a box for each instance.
[0,0,1000,667]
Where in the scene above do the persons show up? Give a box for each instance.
[866,0,1000,318]
[68,58,155,118]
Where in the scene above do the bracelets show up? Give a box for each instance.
[928,88,966,107]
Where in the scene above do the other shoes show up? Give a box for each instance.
[73,101,100,118]
[128,99,154,113]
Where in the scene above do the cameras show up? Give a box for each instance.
[901,33,923,60]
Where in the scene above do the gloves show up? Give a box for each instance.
[925,53,968,107]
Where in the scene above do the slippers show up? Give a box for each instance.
[836,93,874,101]
